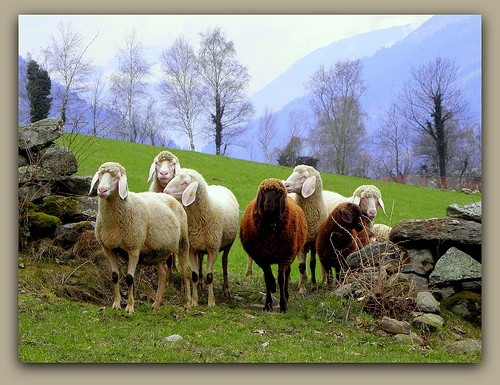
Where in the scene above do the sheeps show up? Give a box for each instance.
[283,165,394,293]
[88,149,240,314]
[240,177,307,314]
